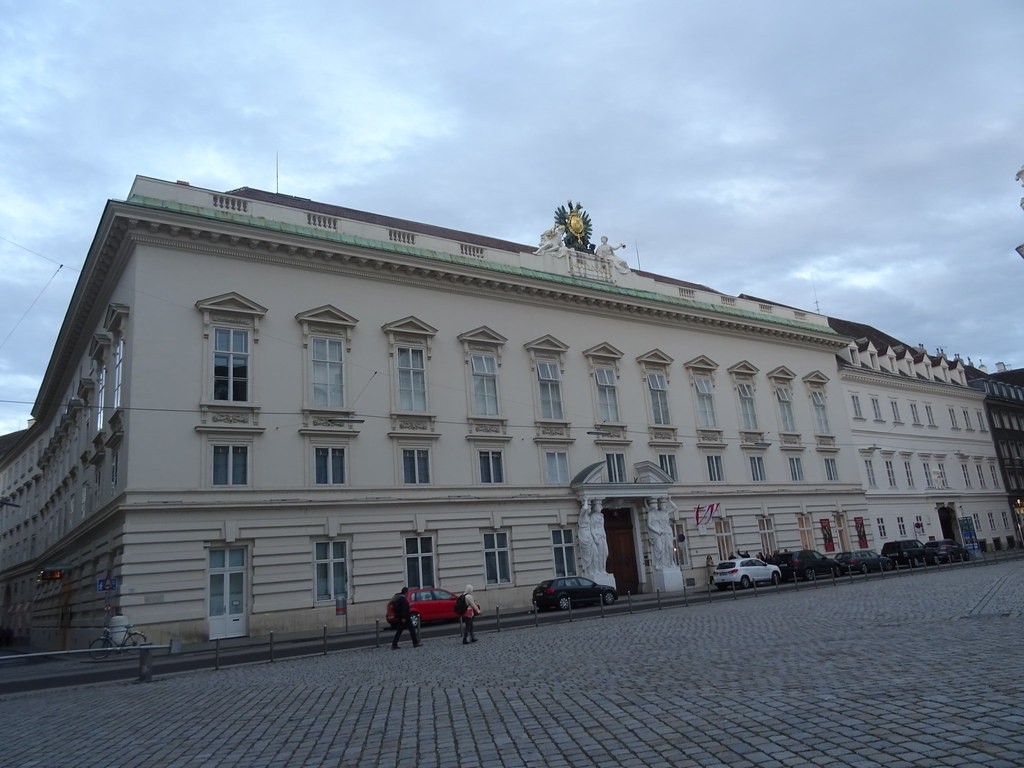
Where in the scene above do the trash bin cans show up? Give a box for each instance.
[110,616,128,655]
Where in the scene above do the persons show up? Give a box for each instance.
[532,225,565,255]
[391,587,423,649]
[737,548,750,558]
[579,504,609,576]
[647,496,677,568]
[729,552,736,560]
[463,585,481,645]
[596,236,627,268]
[756,550,779,564]
[705,556,716,584]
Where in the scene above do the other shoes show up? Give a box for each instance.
[463,641,471,644]
[413,644,422,648]
[471,639,478,642]
[392,645,401,649]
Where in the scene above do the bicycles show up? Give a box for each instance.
[89,624,147,660]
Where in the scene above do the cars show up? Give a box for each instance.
[833,550,894,576]
[925,539,969,564]
[532,576,618,611]
[881,540,939,568]
[386,588,480,628]
[713,558,782,591]
[771,550,843,581]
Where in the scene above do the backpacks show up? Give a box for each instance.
[455,593,470,615]
